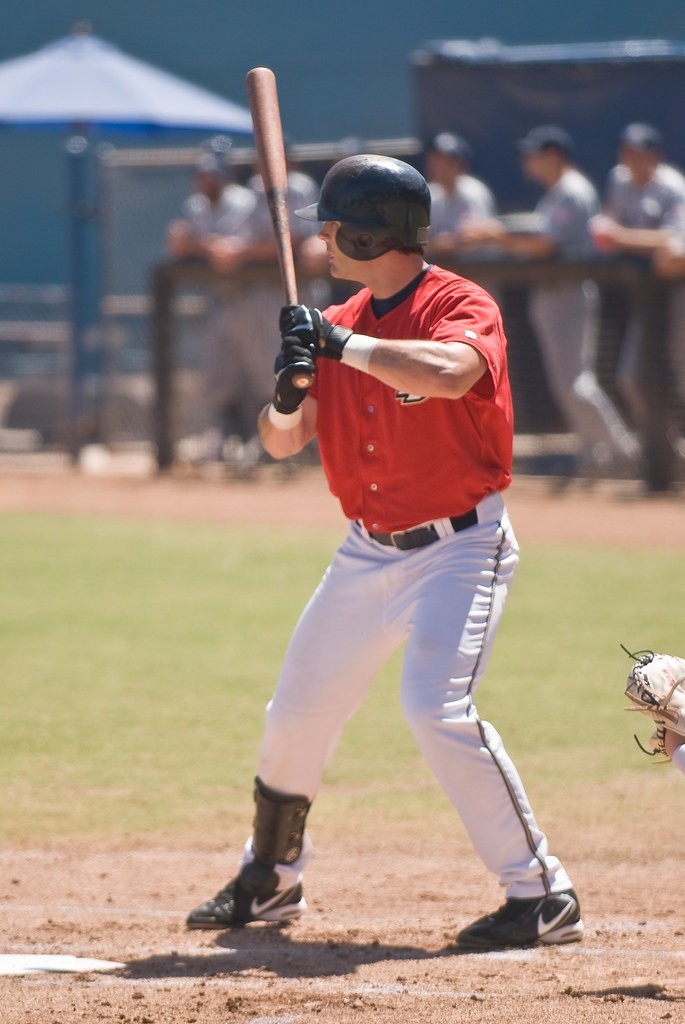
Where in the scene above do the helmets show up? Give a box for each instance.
[294,154,431,261]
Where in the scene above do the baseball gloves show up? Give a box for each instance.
[624,652,685,758]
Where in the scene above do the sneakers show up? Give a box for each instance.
[186,877,308,929]
[455,888,584,948]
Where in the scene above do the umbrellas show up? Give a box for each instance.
[0,30,255,468]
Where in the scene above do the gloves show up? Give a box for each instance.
[279,303,353,362]
[271,336,318,415]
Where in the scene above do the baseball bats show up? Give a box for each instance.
[247,66,316,388]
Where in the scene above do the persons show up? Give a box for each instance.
[170,121,685,491]
[186,154,586,949]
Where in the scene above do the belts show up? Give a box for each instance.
[355,508,478,551]
